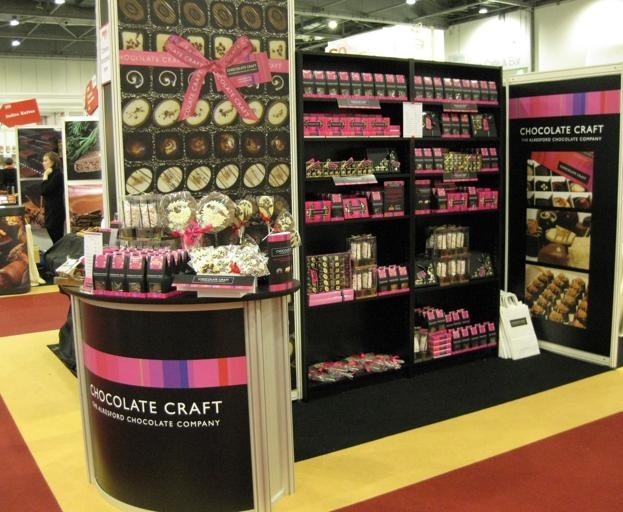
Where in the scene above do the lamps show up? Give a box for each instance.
[406,0,416,6]
[478,7,488,14]
[12,40,21,47]
[328,21,337,30]
[9,16,20,26]
[54,0,66,5]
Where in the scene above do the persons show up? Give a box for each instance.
[1,159,16,192]
[41,151,64,243]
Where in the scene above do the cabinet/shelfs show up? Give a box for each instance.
[295,51,506,402]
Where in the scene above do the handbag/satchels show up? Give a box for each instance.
[498,290,541,362]
